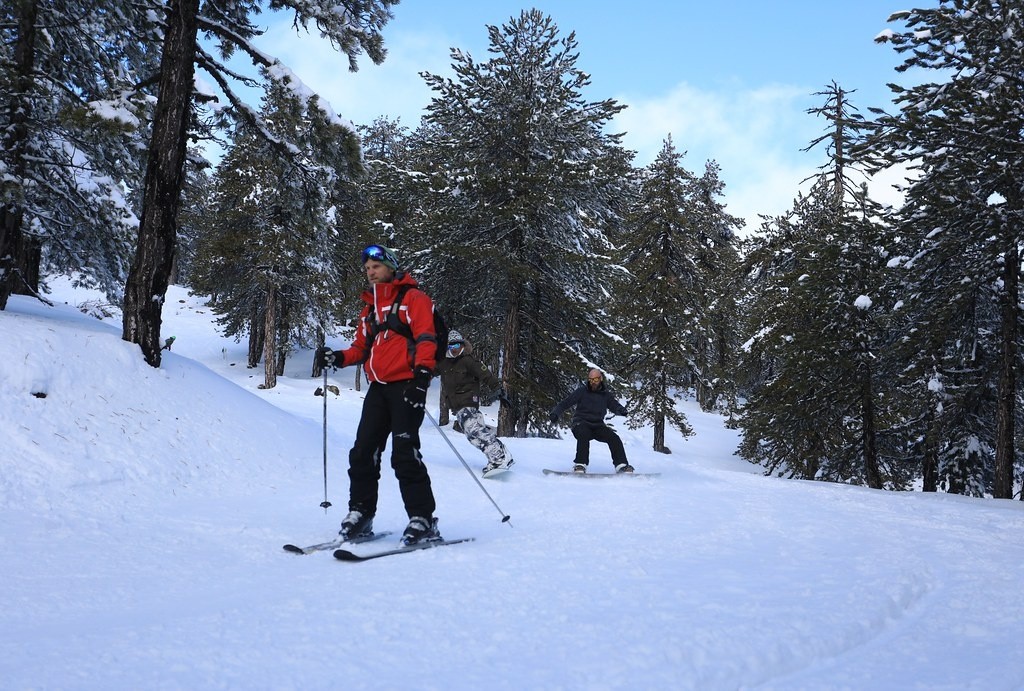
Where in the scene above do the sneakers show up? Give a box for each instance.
[400,514,440,546]
[482,447,514,475]
[338,509,374,540]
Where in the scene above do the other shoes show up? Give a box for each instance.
[574,464,586,474]
[617,463,634,473]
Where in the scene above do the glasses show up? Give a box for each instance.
[447,342,461,350]
[588,375,602,382]
[361,245,385,264]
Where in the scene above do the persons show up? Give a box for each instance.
[315,245,438,550]
[432,330,516,472]
[549,368,634,475]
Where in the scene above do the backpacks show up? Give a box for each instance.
[366,284,448,376]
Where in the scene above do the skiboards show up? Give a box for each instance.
[282,530,476,561]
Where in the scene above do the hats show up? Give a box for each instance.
[377,245,397,271]
[447,330,463,342]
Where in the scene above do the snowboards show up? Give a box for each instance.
[542,469,662,478]
[482,468,511,479]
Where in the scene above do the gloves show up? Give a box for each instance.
[620,408,628,416]
[548,414,558,423]
[404,373,428,407]
[497,390,513,409]
[315,347,345,370]
